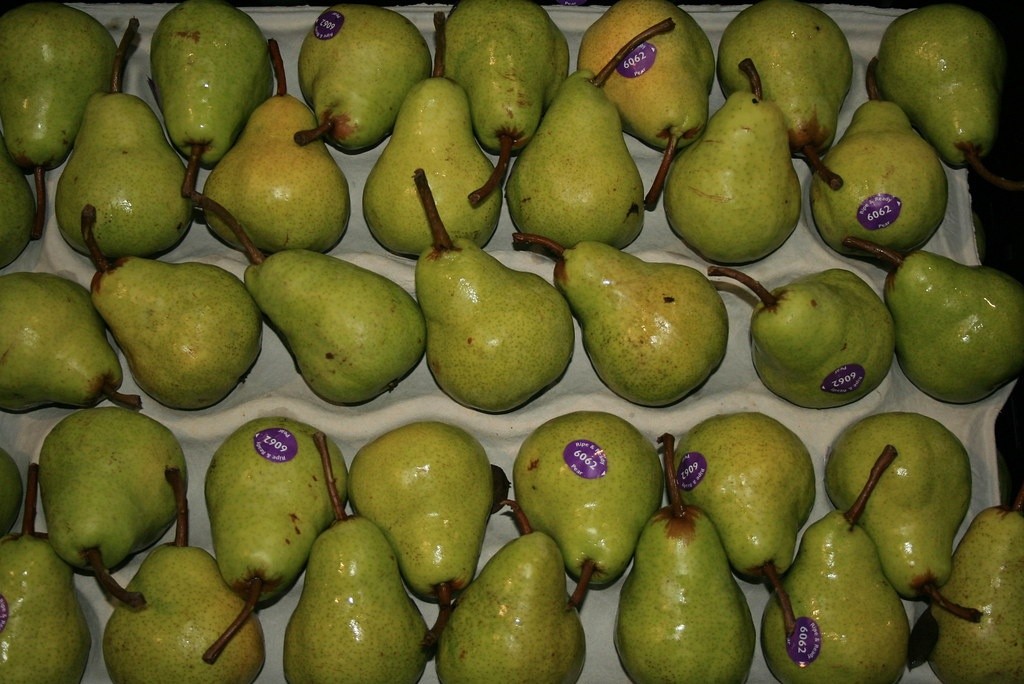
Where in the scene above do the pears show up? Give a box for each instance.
[0,0,1024,684]
